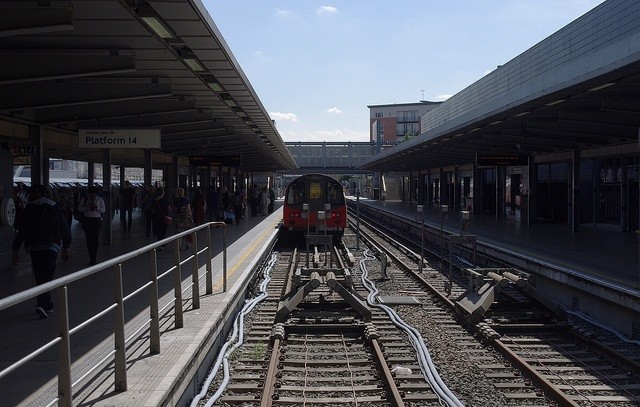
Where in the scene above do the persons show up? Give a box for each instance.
[78,185,106,265]
[134,184,276,251]
[16,184,30,208]
[120,181,135,232]
[11,183,63,318]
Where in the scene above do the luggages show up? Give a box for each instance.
[224,207,235,224]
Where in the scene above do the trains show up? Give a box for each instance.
[282,172,347,247]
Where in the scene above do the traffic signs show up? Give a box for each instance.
[10,143,37,156]
[76,128,161,149]
[474,150,530,166]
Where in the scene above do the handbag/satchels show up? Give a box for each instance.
[219,206,226,219]
[74,194,88,221]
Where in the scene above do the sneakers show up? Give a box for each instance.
[47,302,53,312]
[36,304,48,318]
